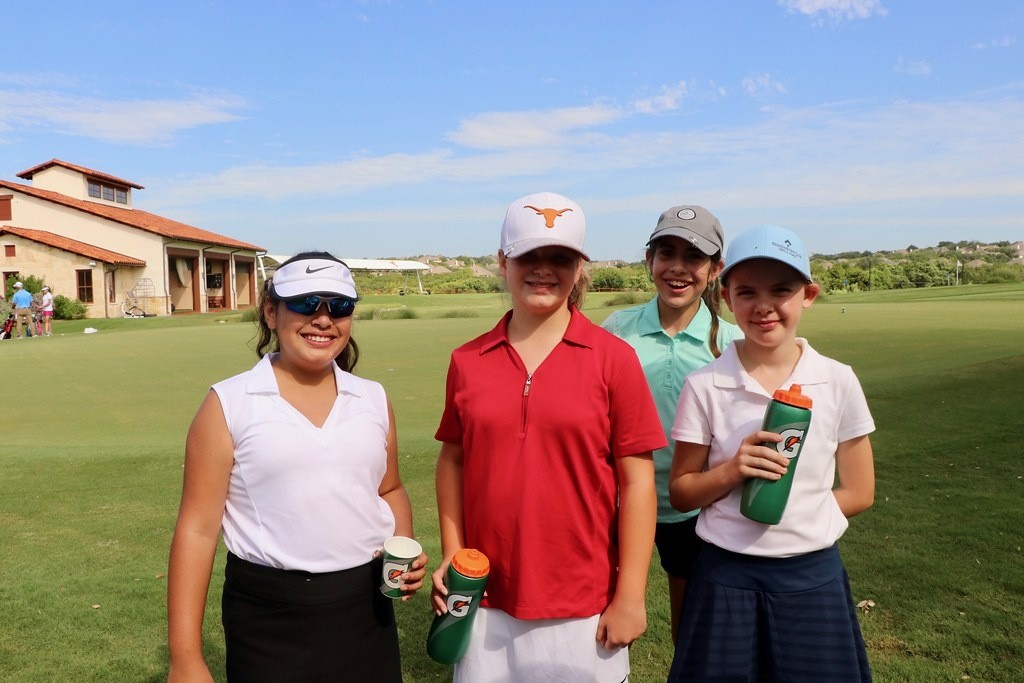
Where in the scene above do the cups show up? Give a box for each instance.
[380,535,423,599]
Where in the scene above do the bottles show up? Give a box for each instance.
[426,548,490,663]
[739,385,813,524]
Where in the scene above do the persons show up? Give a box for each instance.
[598,205,745,645]
[667,223,876,683]
[167,251,429,683]
[12,282,37,338]
[431,191,668,683]
[39,286,53,336]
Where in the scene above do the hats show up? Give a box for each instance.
[267,258,358,301]
[646,205,724,263]
[501,191,590,263]
[719,225,814,287]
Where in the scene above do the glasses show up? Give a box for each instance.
[275,292,356,319]
